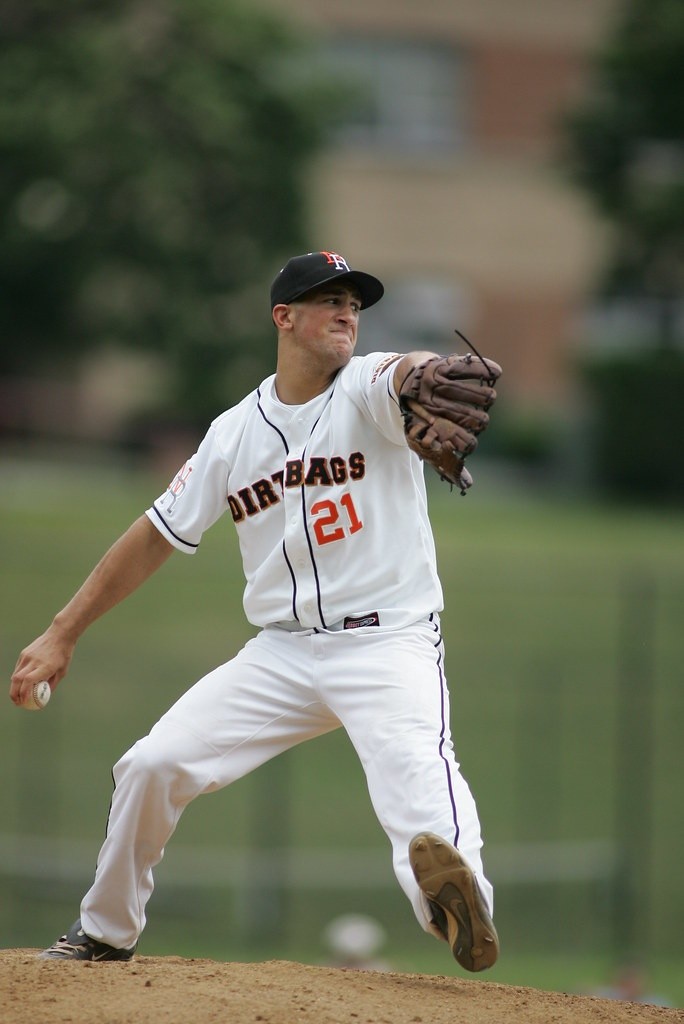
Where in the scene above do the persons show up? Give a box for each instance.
[11,248,502,972]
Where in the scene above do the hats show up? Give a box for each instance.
[271,251,385,317]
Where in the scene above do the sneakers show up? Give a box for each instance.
[409,830,499,973]
[38,919,137,962]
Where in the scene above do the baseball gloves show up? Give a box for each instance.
[396,327,502,498]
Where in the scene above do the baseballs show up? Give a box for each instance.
[20,680,52,711]
